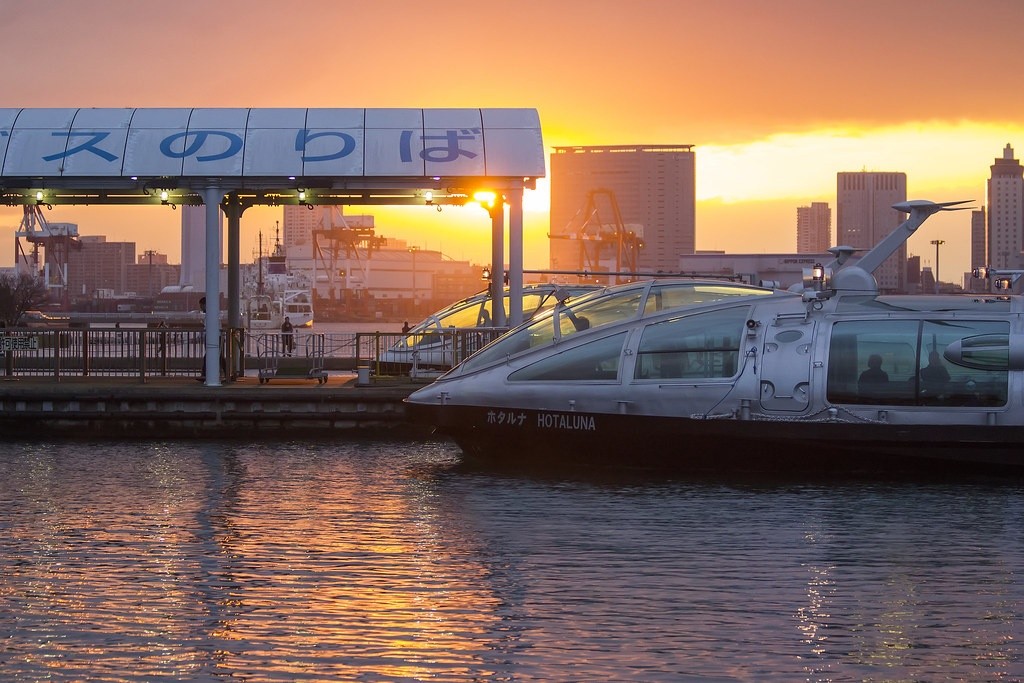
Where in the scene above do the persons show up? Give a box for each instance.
[566,316,598,379]
[402,321,416,335]
[962,380,982,406]
[920,352,951,407]
[196,297,227,384]
[478,309,493,346]
[857,355,889,406]
[282,317,294,357]
[157,321,168,357]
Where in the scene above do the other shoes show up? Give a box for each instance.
[195,377,206,382]
[221,376,233,384]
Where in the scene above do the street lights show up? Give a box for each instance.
[142,250,157,312]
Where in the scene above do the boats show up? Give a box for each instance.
[338,283,604,402]
[284,288,314,327]
[243,229,284,329]
[400,279,1023,479]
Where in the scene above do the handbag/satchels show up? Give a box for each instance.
[291,337,296,349]
